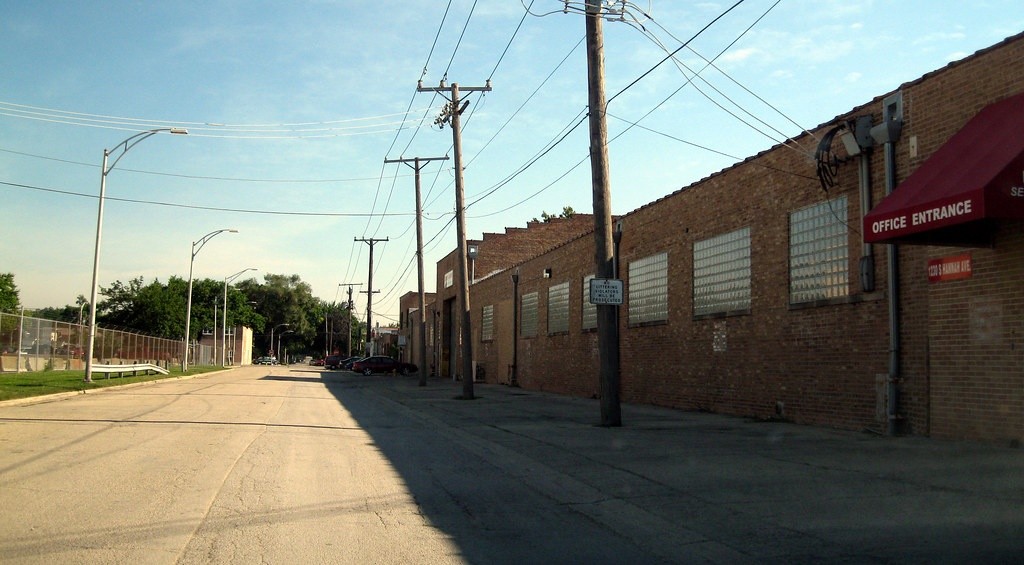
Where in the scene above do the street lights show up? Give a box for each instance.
[271,324,290,365]
[185,228,239,370]
[84,128,187,382]
[221,268,258,367]
[277,331,293,363]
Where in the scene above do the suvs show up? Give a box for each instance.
[324,356,340,370]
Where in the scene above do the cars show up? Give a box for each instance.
[352,355,418,376]
[341,356,363,368]
